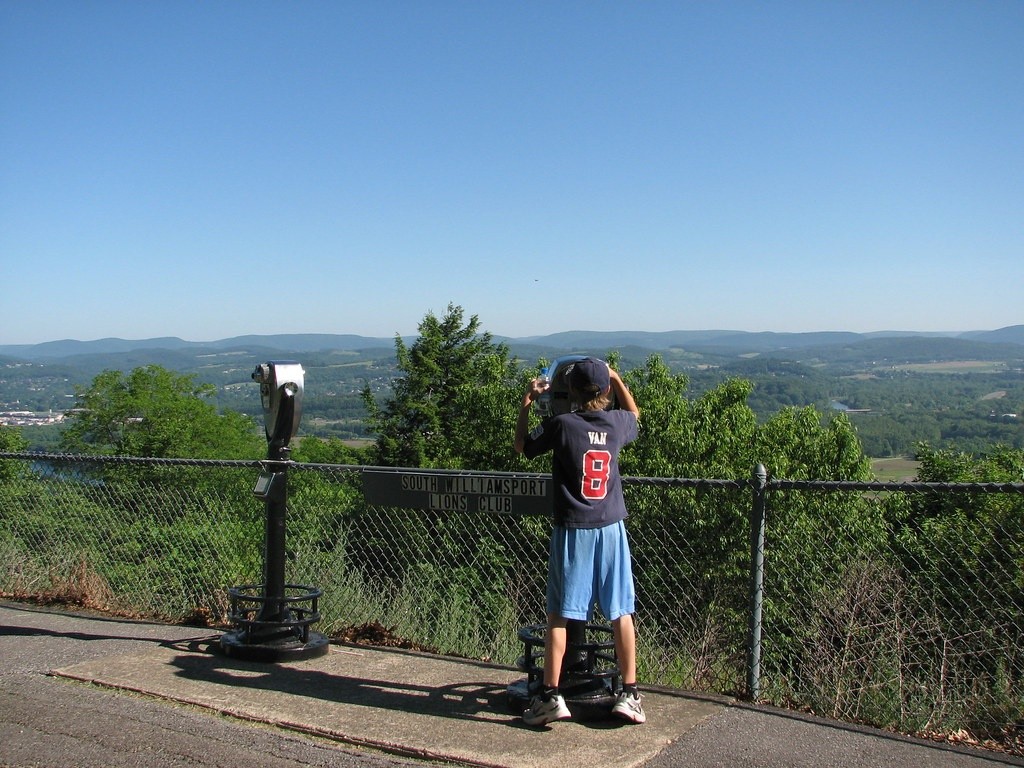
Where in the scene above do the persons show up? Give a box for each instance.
[514,356,647,726]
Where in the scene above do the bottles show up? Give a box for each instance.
[534,368,551,416]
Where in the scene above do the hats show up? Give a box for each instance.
[569,357,610,397]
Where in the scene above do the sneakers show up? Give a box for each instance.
[612,692,646,723]
[522,693,571,726]
